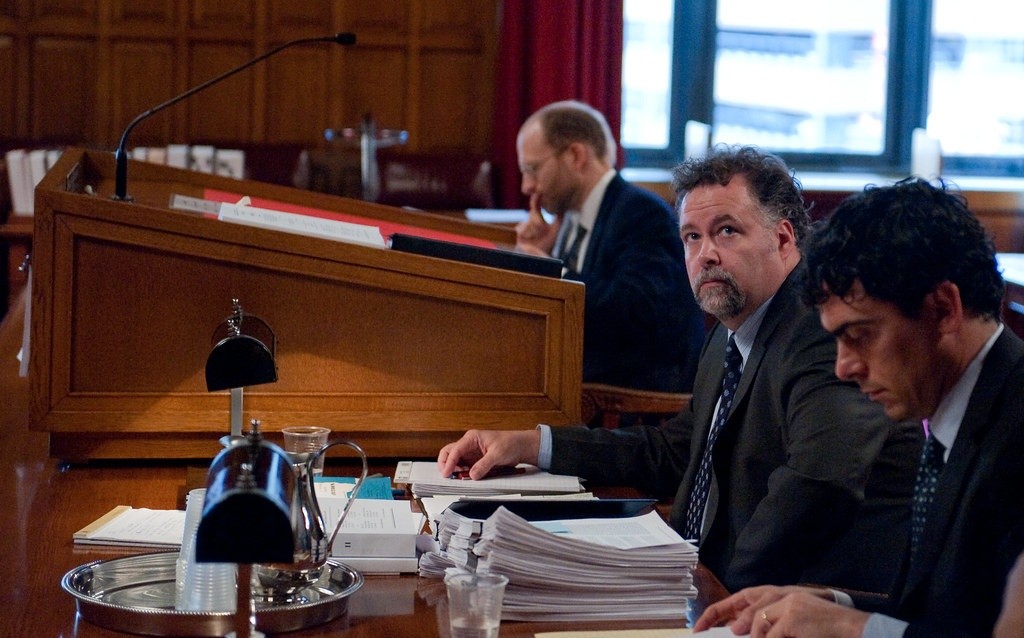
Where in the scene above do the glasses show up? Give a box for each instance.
[521,141,575,178]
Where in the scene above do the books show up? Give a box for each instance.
[313,476,395,501]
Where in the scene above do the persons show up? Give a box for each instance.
[691,177,1024,638]
[436,143,927,609]
[510,101,705,430]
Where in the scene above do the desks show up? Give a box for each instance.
[1,280,736,638]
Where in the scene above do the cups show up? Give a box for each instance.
[443,573,509,638]
[175,488,238,613]
[281,425,330,482]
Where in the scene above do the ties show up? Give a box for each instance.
[563,223,587,277]
[898,425,944,607]
[680,336,742,546]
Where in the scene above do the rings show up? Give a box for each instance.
[760,609,773,625]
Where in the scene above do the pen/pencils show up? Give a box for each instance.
[392,489,406,498]
[451,467,527,480]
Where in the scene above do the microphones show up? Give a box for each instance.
[112,31,356,203]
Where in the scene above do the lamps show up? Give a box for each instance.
[194,296,299,638]
